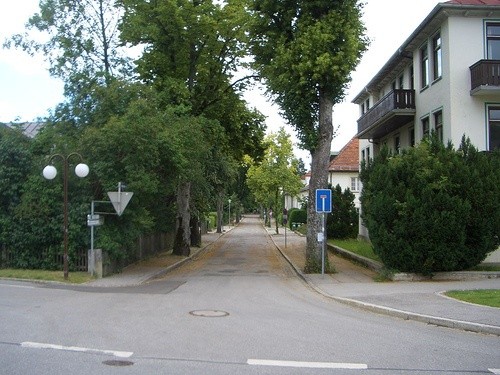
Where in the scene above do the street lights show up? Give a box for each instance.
[43,150,88,281]
